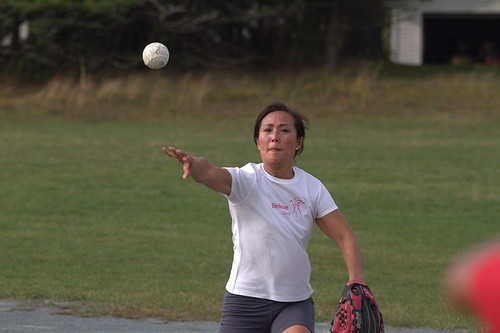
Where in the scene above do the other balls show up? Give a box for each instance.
[142,42,169,69]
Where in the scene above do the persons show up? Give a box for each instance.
[163,102,363,333]
